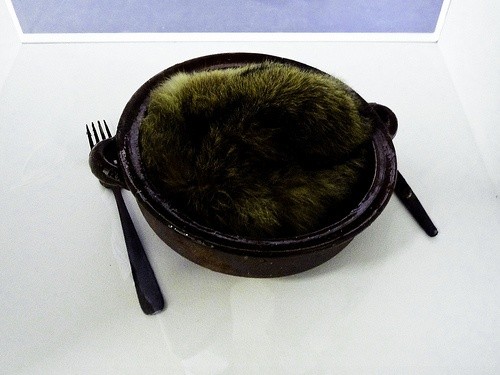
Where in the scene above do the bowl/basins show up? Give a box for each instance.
[90,53,398,279]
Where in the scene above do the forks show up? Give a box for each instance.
[86,120,165,316]
